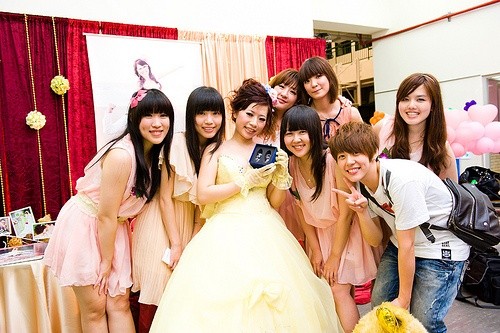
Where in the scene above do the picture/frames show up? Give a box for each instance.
[0,206,56,241]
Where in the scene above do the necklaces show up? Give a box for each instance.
[304,177,311,183]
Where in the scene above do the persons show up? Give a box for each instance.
[280,104,378,333]
[131,86,226,333]
[378,71,459,186]
[22,211,34,234]
[298,56,363,150]
[330,122,470,333]
[45,90,174,333]
[260,68,352,146]
[0,219,9,233]
[149,78,345,333]
[134,59,162,91]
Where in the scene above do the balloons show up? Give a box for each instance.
[370,111,385,126]
[444,100,500,157]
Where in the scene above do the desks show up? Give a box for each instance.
[0,249,84,333]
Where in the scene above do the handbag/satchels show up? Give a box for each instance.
[455,247,500,308]
[442,177,500,247]
[458,166,500,196]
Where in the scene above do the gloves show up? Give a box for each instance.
[235,162,277,197]
[270,149,293,190]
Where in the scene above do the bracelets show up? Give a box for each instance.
[193,221,204,228]
